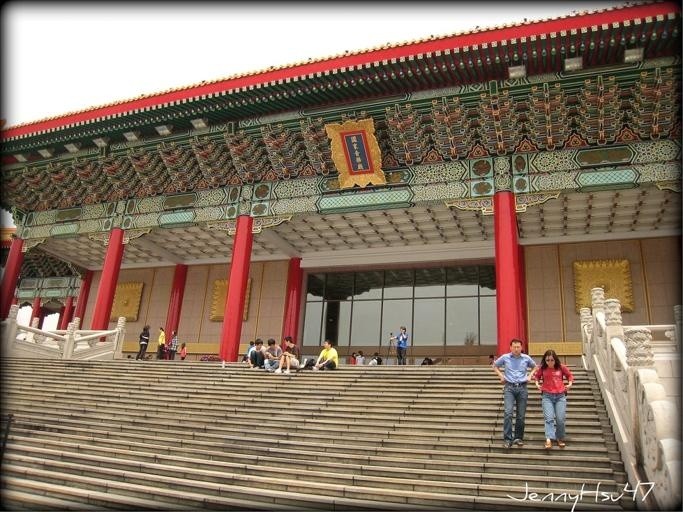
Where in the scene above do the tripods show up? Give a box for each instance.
[386,333,401,365]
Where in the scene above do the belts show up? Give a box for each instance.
[512,382,521,387]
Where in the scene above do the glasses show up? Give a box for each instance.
[546,359,554,362]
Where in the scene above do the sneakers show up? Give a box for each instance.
[284,369,290,373]
[275,368,282,373]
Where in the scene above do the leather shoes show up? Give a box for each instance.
[544,440,553,448]
[502,442,510,449]
[557,439,565,447]
[515,440,524,445]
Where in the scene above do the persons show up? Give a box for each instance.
[535,351,574,451]
[488,355,497,366]
[242,336,340,375]
[492,339,536,450]
[349,351,381,366]
[128,325,187,363]
[389,326,408,365]
[421,358,433,365]
